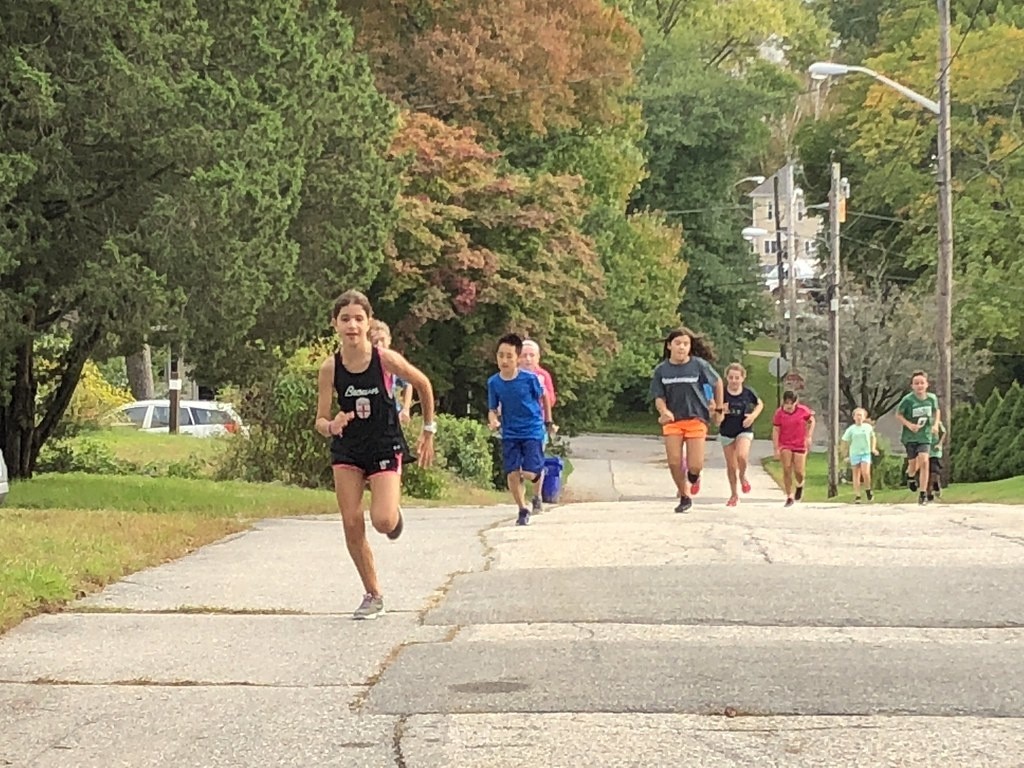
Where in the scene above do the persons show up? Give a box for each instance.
[651,330,724,513]
[905,421,947,492]
[841,407,879,504]
[895,369,941,504]
[719,362,763,506]
[772,391,815,507]
[487,333,560,525]
[519,338,556,510]
[315,290,437,618]
[366,319,413,429]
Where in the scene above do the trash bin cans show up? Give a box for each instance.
[541,456,564,503]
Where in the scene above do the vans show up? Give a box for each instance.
[102,400,249,443]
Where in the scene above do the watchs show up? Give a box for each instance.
[424,422,437,434]
[714,409,723,414]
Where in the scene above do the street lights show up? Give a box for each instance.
[810,0,952,489]
[742,162,842,498]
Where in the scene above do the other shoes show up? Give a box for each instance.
[516,507,531,525]
[784,498,794,506]
[794,487,803,500]
[531,472,541,484]
[688,470,699,483]
[907,473,917,492]
[727,495,738,506]
[933,481,940,492]
[866,489,874,500]
[928,494,935,500]
[675,496,691,512]
[532,495,543,513]
[918,491,928,504]
[741,480,751,493]
[691,481,701,494]
[855,495,862,504]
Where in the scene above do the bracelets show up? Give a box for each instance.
[545,420,555,427]
[329,421,343,437]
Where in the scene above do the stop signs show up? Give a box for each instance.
[783,374,807,396]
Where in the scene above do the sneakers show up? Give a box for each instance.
[352,593,386,620]
[386,505,404,540]
